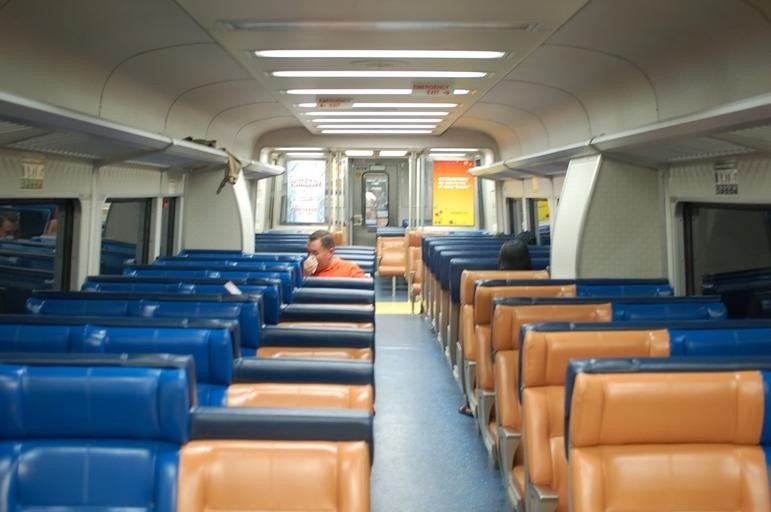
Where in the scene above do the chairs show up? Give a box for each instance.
[152,223,379,509]
[1,264,152,511]
[0,230,376,512]
[376,225,408,298]
[407,230,427,314]
[0,235,134,312]
[421,228,770,510]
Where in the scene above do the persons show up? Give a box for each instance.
[303,229,368,278]
[0,212,21,241]
[45,217,57,236]
[497,239,532,269]
[515,232,536,244]
[365,192,377,219]
